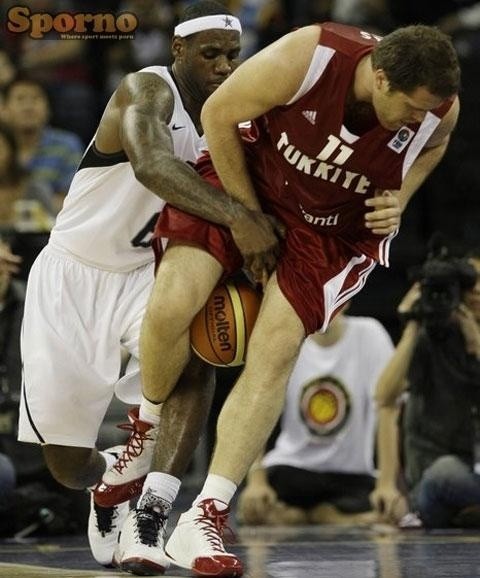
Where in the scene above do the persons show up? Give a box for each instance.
[0,0,480,578]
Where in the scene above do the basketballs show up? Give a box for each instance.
[188,280,261,366]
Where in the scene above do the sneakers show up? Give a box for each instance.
[94,406,161,506]
[164,498,243,576]
[89,446,132,568]
[113,491,170,574]
